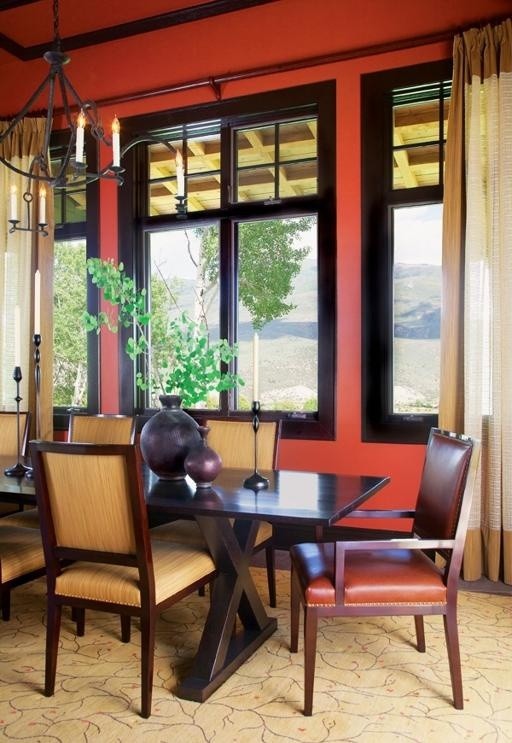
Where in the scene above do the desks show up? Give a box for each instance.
[0,454,391,703]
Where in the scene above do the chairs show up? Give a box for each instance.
[290,427,482,716]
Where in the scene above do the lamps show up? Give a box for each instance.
[0,0,189,237]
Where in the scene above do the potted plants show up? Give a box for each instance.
[79,257,244,480]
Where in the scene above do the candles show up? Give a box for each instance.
[35,270,40,334]
[14,304,20,367]
[252,332,259,401]
